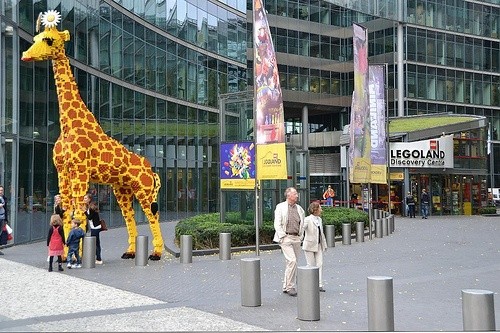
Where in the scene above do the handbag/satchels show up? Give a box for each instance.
[6,223,13,240]
[99,220,108,232]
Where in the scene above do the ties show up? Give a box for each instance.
[318,226,321,243]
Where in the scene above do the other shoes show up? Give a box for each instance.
[425,217,427,219]
[59,264,64,272]
[0,250,4,255]
[67,262,82,268]
[422,217,424,219]
[95,259,103,265]
[284,288,297,296]
[320,287,325,292]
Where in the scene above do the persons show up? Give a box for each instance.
[0,185,9,255]
[79,193,103,265]
[301,201,328,292]
[272,187,305,296]
[66,219,84,269]
[54,194,65,221]
[47,214,65,272]
[406,191,416,218]
[323,185,335,207]
[420,188,429,219]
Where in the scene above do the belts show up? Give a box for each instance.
[286,232,298,235]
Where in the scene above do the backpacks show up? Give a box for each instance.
[50,226,63,250]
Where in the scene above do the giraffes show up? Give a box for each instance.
[21,8,167,263]
